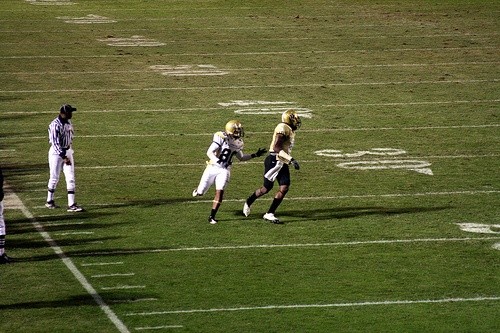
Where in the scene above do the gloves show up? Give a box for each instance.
[251,148,266,158]
[292,161,300,170]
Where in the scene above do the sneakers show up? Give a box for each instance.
[264,213,278,221]
[192,188,198,197]
[242,203,250,216]
[45,201,55,209]
[209,215,217,226]
[67,204,82,212]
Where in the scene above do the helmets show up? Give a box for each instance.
[281,109,299,129]
[225,119,241,137]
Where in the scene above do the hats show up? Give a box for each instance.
[60,105,76,112]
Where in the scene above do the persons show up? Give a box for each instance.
[243,108,300,222]
[44,104,85,212]
[0,163,12,262]
[193,120,266,224]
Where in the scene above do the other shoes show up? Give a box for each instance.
[0,254,12,263]
